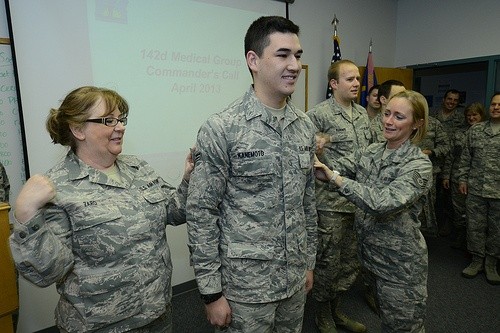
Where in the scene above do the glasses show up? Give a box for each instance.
[83,117,128,127]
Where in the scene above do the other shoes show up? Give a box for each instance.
[461,255,483,278]
[313,301,337,333]
[333,298,367,332]
[484,256,500,284]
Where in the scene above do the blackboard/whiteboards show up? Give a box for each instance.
[0,38,31,210]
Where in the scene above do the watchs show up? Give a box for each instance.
[330,170,340,184]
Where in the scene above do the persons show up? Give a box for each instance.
[422,102,486,252]
[370,80,407,144]
[366,85,381,119]
[311,89,433,333]
[413,115,452,245]
[189,14,319,333]
[0,162,20,333]
[454,90,500,285]
[8,85,196,333]
[434,89,468,132]
[305,60,371,333]
